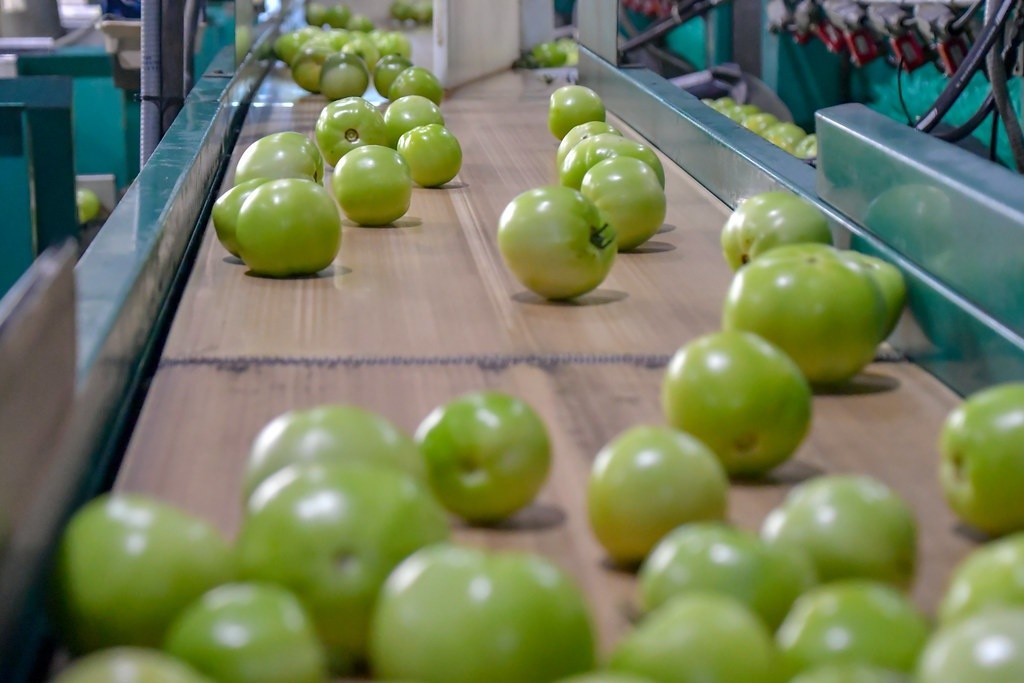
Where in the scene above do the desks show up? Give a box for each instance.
[0,44,141,295]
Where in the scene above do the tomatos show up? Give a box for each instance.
[55,0,1024,683]
